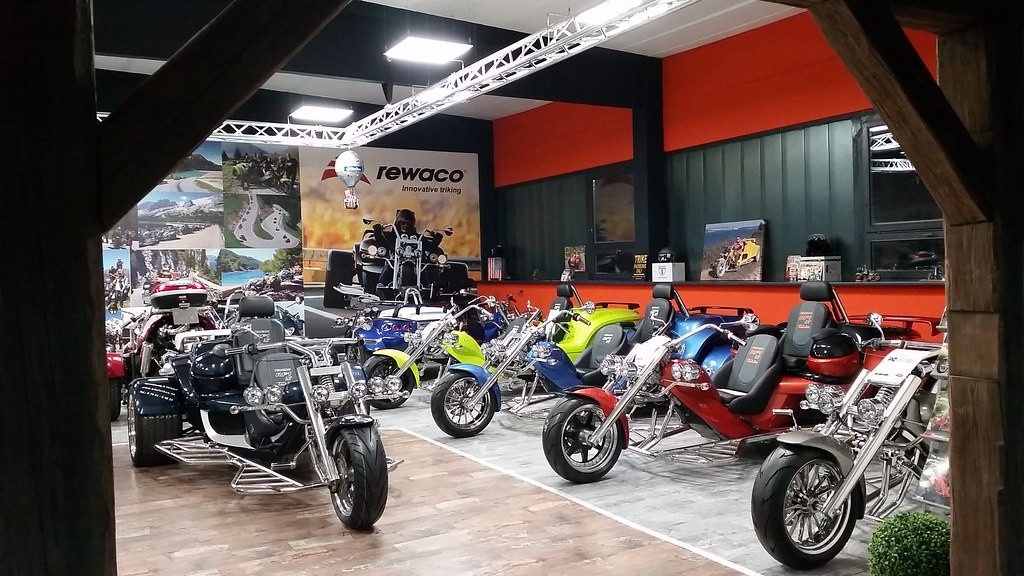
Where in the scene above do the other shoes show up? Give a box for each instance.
[376,283,387,300]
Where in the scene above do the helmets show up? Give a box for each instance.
[397,209,415,223]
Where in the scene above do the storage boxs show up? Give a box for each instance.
[799,256,841,281]
[652,262,685,282]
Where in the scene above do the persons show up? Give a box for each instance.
[109,266,117,280]
[374,209,444,298]
[732,236,744,268]
[116,258,124,269]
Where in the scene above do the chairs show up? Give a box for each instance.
[232,296,286,387]
[542,284,574,341]
[497,316,527,339]
[756,280,835,371]
[629,282,675,347]
[710,334,784,414]
[249,352,302,426]
[574,323,627,386]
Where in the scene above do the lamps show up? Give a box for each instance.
[383,0,474,66]
[288,92,354,123]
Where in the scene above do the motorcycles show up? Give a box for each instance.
[99,281,954,571]
[231,156,293,195]
[715,237,761,278]
[323,215,470,318]
[855,265,880,282]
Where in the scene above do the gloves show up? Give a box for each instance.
[373,223,381,231]
[436,232,443,240]
